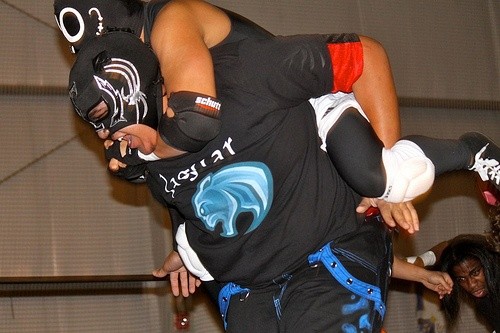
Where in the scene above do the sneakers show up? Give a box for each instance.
[460,131,500,193]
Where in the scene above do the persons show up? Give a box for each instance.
[53,2,500,333]
